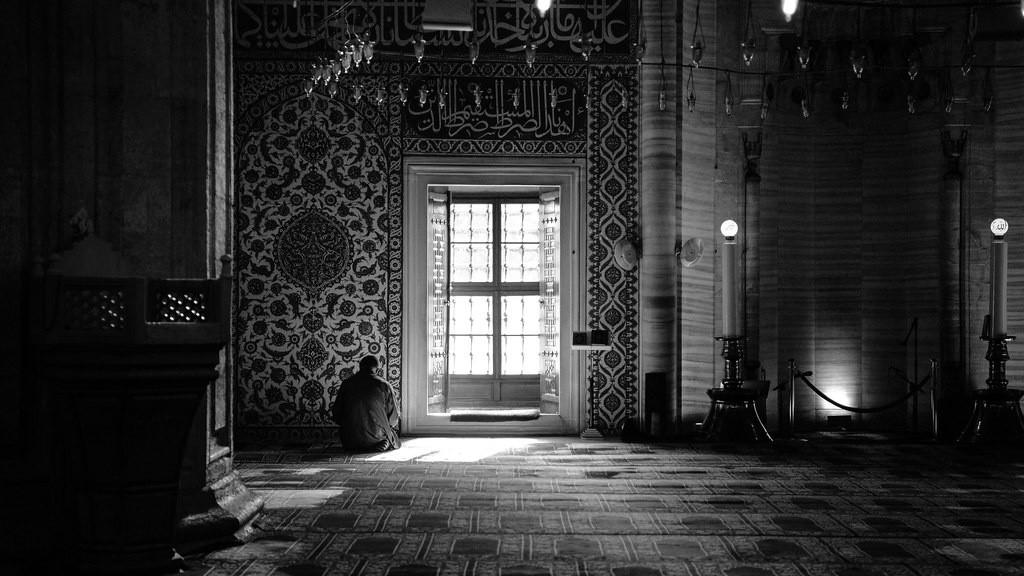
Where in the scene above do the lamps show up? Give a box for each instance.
[952,217,1024,455]
[701,220,775,447]
[299,0,995,119]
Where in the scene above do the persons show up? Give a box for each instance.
[332,355,399,451]
